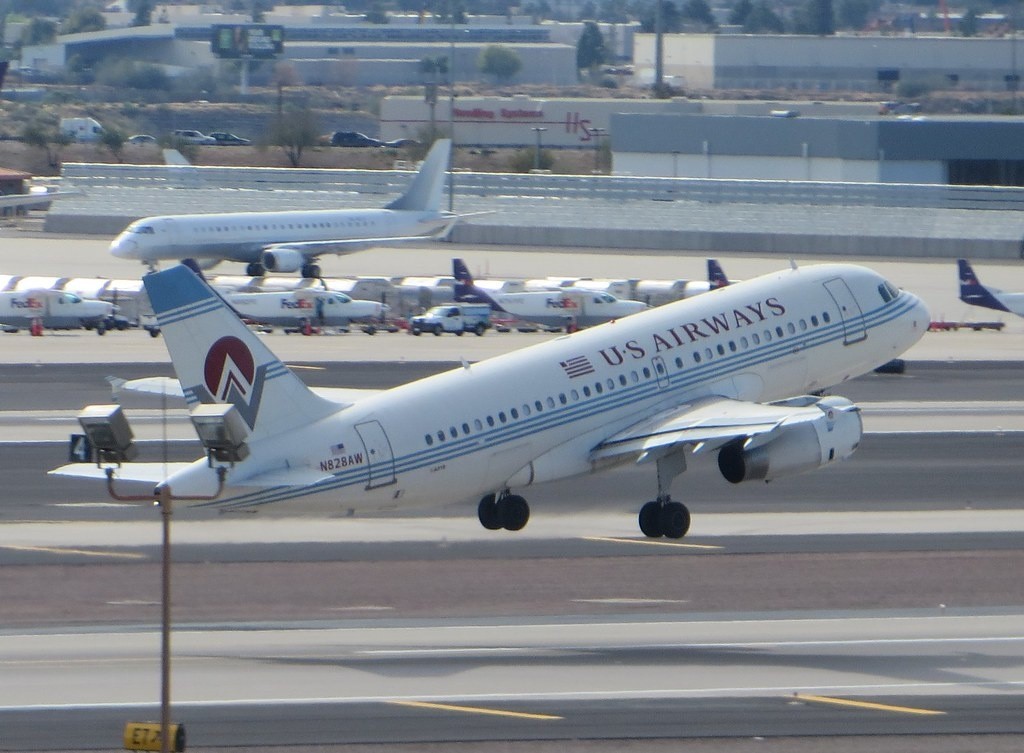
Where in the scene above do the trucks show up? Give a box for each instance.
[415,300,492,339]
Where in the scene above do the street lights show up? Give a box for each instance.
[78,405,251,752]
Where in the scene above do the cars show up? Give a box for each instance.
[125,136,158,144]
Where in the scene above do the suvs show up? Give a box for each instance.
[168,131,246,146]
[330,131,424,147]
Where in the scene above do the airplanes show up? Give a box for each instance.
[107,140,509,279]
[0,255,935,541]
[955,258,1024,318]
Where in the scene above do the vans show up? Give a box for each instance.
[55,117,107,139]
